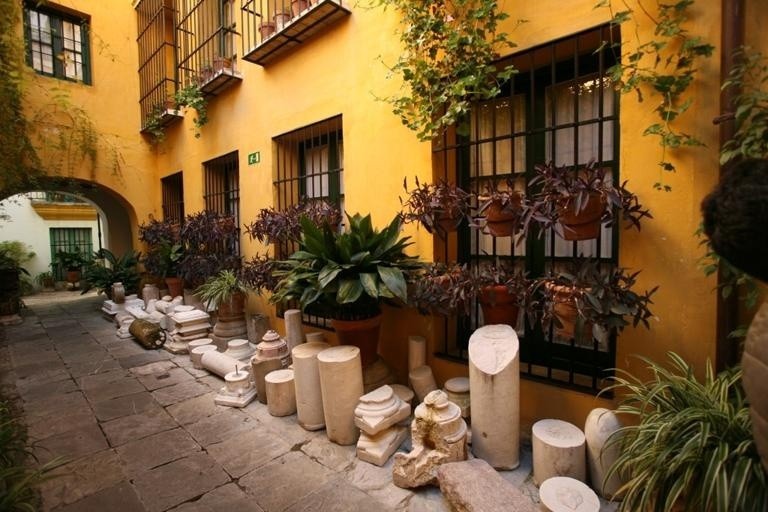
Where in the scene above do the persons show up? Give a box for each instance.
[701,154,766,470]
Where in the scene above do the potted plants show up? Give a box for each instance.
[80,247,145,300]
[34,270,56,288]
[138,211,251,323]
[435,263,476,317]
[56,245,84,282]
[595,346,767,512]
[533,167,651,241]
[536,263,658,343]
[401,177,473,236]
[476,268,526,330]
[271,210,428,364]
[478,184,532,241]
